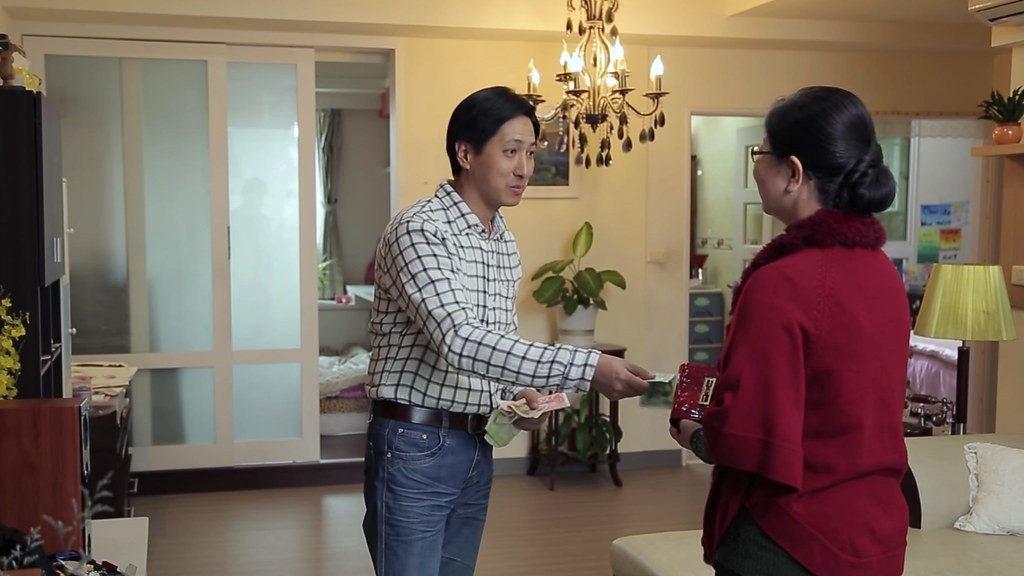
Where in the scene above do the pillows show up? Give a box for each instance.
[954,443,1024,536]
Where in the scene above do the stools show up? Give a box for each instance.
[529,343,626,490]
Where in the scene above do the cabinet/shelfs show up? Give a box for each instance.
[70,363,139,520]
[690,288,727,365]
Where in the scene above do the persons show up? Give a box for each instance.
[362,86,656,575]
[669,86,911,576]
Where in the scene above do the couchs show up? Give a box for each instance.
[903,432,1024,576]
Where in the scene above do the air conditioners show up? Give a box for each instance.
[968,0,1024,27]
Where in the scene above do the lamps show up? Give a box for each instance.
[914,264,1017,432]
[521,0,669,169]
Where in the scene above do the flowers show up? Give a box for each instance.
[0,287,31,401]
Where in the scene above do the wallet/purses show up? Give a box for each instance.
[668,361,728,432]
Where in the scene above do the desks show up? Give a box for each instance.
[82,516,151,576]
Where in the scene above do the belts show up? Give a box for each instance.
[371,397,486,436]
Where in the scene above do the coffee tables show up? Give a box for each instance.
[609,530,715,576]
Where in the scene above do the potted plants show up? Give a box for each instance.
[976,85,1024,144]
[530,222,627,346]
[541,393,621,469]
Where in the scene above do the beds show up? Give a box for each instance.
[318,342,374,436]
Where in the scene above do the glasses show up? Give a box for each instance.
[750,145,775,158]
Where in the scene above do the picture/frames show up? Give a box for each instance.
[521,104,580,199]
